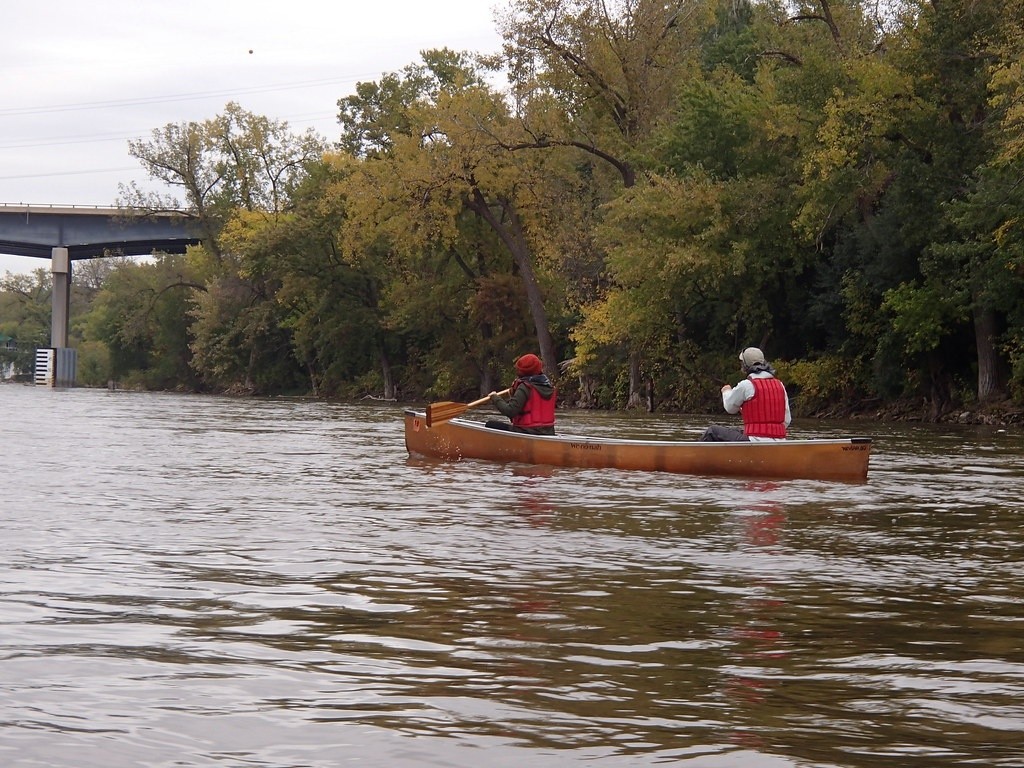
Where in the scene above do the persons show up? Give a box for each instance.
[699,347,791,442]
[485,354,557,436]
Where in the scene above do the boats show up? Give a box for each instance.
[403,408,874,483]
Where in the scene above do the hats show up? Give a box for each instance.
[516,354,542,377]
[739,347,765,368]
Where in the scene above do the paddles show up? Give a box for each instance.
[425,386,512,429]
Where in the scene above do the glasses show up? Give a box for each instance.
[742,347,747,366]
